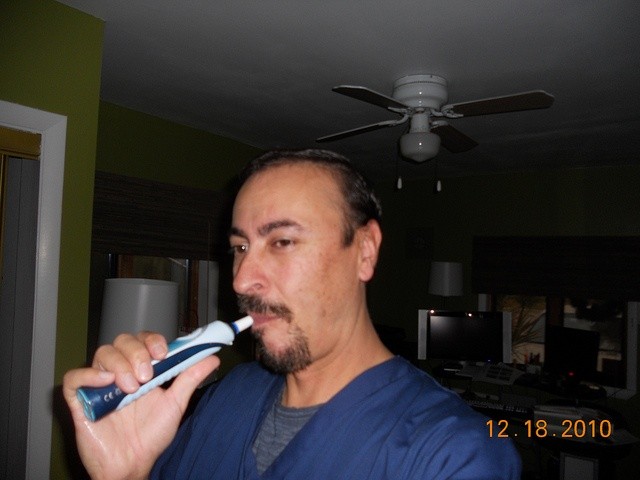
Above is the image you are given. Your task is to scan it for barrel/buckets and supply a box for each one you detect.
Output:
[96,278,179,349]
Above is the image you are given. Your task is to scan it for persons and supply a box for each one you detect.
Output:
[62,150,521,480]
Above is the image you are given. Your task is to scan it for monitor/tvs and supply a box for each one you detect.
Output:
[426,310,503,371]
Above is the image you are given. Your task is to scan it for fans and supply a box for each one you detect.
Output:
[315,75,555,160]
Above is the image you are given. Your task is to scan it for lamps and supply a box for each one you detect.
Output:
[427,260,466,309]
[397,116,442,163]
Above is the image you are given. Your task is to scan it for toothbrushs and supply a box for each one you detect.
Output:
[75,315,254,422]
